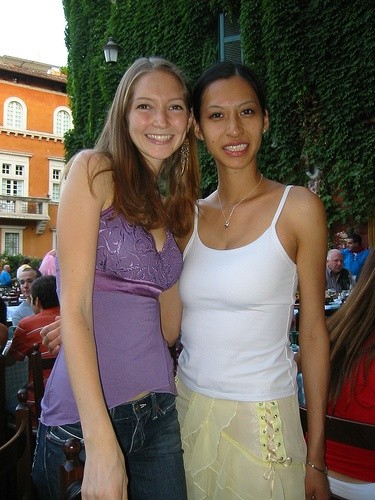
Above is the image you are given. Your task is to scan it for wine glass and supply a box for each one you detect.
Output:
[328,288,336,302]
[348,275,356,289]
[343,290,350,302]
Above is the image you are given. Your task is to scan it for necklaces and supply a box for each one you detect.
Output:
[215,169,264,229]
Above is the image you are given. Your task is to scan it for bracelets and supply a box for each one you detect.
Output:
[305,456,328,479]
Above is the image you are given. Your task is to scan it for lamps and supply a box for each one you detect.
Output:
[103,37,119,64]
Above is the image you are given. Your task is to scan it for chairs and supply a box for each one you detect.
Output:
[60,437,84,500]
[0,389,36,500]
[298,404,375,500]
[29,344,74,425]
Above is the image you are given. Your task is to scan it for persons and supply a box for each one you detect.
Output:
[0,255,10,274]
[37,248,58,278]
[0,297,9,351]
[291,247,375,500]
[39,315,64,355]
[340,234,370,282]
[16,264,32,282]
[157,58,332,500]
[27,54,201,500]
[0,264,18,289]
[325,248,356,292]
[9,267,45,328]
[3,273,62,430]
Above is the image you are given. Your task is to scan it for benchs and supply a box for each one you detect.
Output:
[0,286,21,307]
